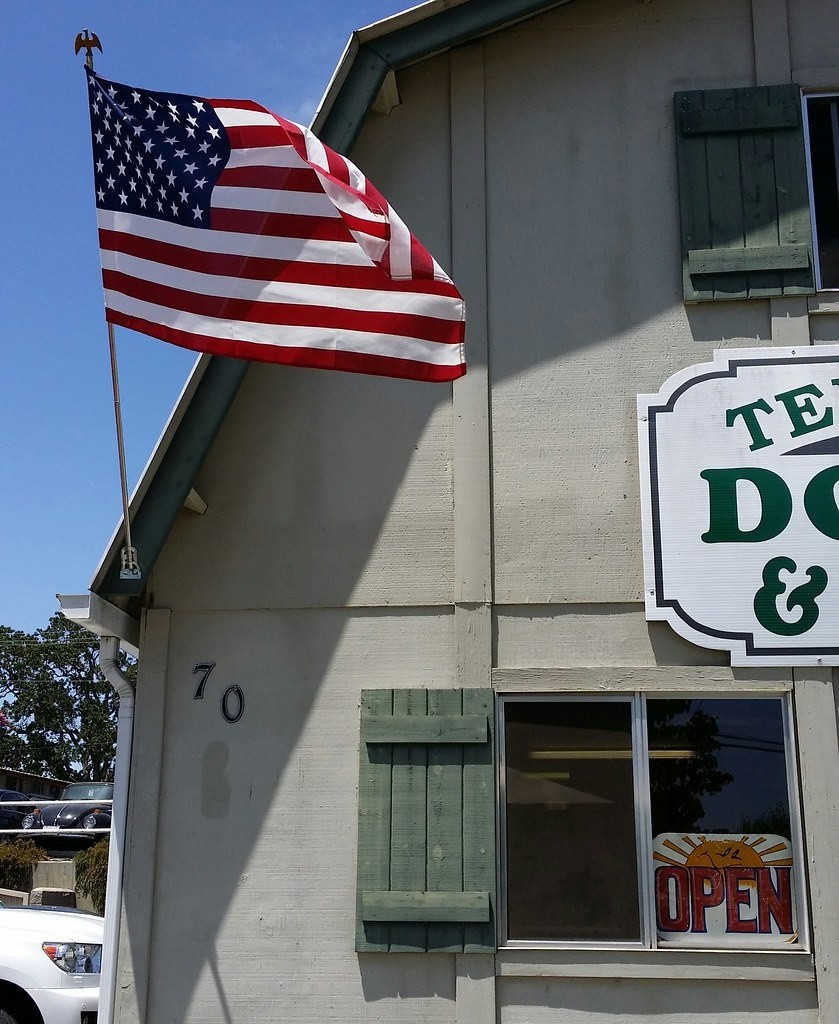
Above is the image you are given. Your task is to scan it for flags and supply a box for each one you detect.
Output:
[82,68,470,383]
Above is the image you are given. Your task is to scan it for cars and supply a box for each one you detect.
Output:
[0,899,108,1024]
[0,789,38,829]
[22,781,116,830]
